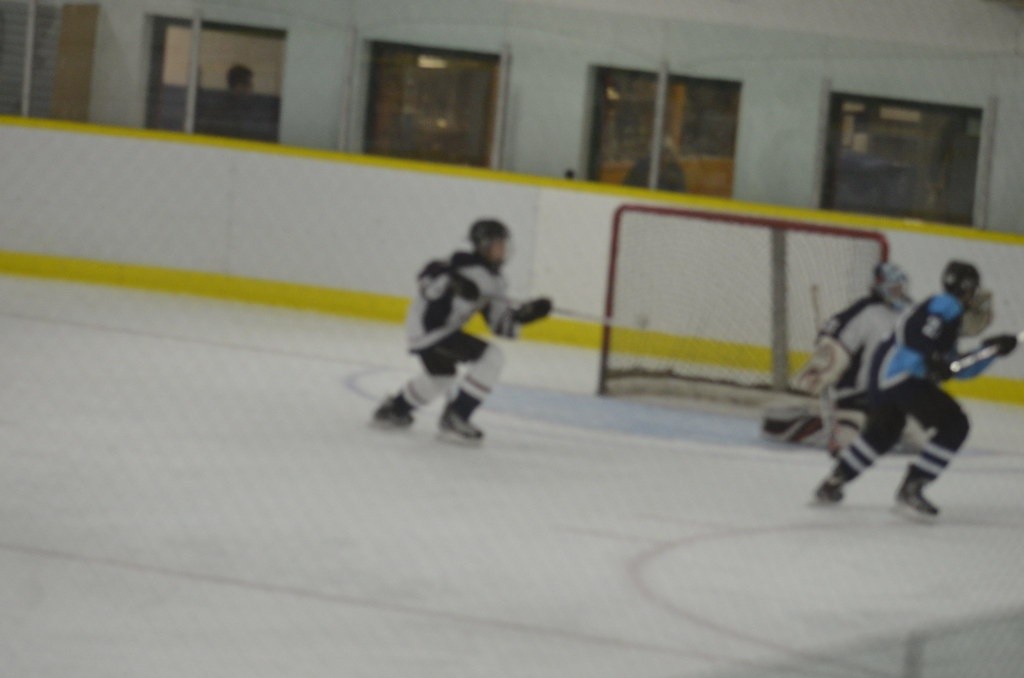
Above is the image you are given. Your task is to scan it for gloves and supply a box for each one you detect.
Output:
[984,335,1018,355]
[928,349,953,372]
[518,299,549,322]
[451,272,479,300]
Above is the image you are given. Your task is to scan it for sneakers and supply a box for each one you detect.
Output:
[816,485,843,505]
[375,405,412,427]
[438,409,483,445]
[896,490,937,518]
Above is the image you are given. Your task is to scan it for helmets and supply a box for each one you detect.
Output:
[471,221,504,253]
[943,261,979,296]
[871,263,907,304]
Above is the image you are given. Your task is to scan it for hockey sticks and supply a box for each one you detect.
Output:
[476,293,651,333]
[808,282,843,458]
[950,331,1024,373]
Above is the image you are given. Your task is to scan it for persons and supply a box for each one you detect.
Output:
[628,139,688,196]
[366,218,556,442]
[203,64,266,142]
[764,257,1019,517]
